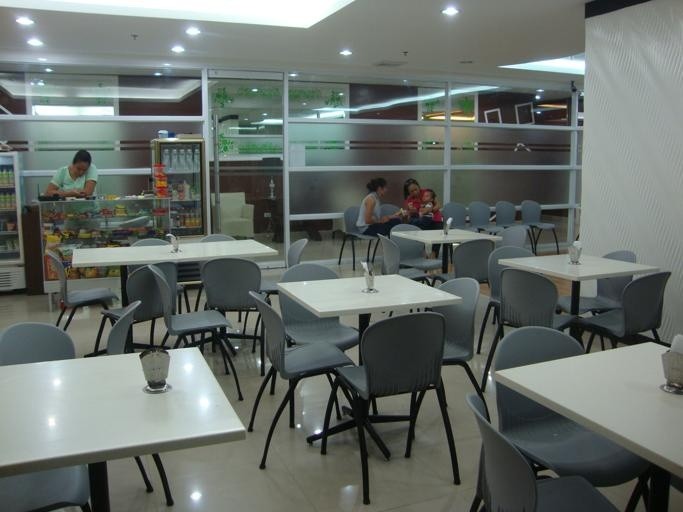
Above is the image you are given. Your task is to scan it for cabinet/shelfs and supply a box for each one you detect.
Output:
[38,193,170,282]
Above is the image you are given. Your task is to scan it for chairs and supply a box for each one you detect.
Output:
[378,204,400,217]
[199,258,272,352]
[147,263,244,401]
[477,247,541,355]
[248,288,396,469]
[337,204,380,271]
[430,240,496,288]
[479,269,584,391]
[390,224,443,283]
[125,237,190,314]
[465,393,622,512]
[556,249,638,353]
[91,261,176,353]
[577,271,671,353]
[374,232,433,287]
[494,201,533,254]
[467,201,503,237]
[105,300,142,356]
[491,324,657,512]
[242,238,309,353]
[277,261,363,430]
[318,312,462,505]
[0,321,95,512]
[402,275,492,485]
[47,247,118,332]
[440,201,478,263]
[519,199,559,253]
[193,234,246,323]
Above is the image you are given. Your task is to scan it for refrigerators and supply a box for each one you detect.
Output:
[149,136,207,240]
[0,151,27,291]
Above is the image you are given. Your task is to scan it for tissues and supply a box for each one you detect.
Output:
[567,240,583,265]
[359,261,378,293]
[443,218,452,233]
[166,233,182,253]
[659,333,683,395]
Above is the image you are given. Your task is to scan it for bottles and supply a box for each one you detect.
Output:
[194,148,201,171]
[171,207,201,228]
[0,168,3,184]
[171,148,178,171]
[5,192,11,209]
[186,148,194,171]
[178,148,186,171]
[3,168,9,184]
[11,193,16,209]
[0,191,5,208]
[8,168,14,185]
[163,148,170,170]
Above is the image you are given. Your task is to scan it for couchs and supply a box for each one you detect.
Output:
[210,190,255,238]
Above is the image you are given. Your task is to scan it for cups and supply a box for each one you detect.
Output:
[566,246,583,266]
[170,236,182,253]
[658,350,682,396]
[361,270,378,293]
[444,225,449,236]
[139,348,172,394]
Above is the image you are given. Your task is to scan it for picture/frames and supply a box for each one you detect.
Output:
[483,108,502,123]
[514,102,534,124]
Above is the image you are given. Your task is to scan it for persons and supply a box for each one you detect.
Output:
[399,178,444,259]
[401,189,436,219]
[46,149,99,203]
[355,177,402,239]
